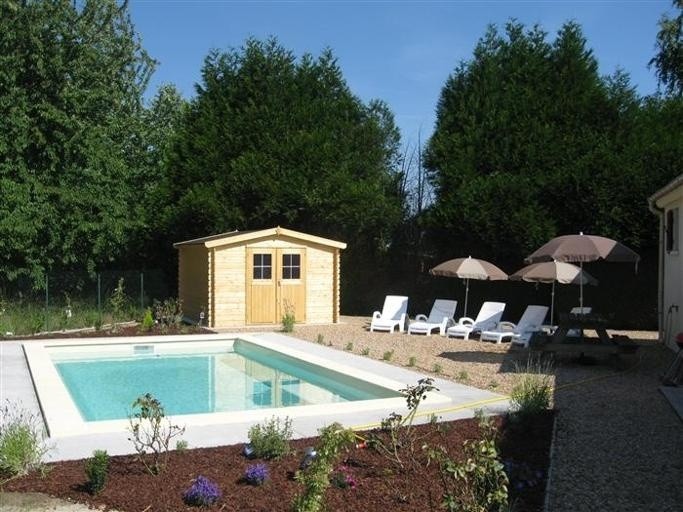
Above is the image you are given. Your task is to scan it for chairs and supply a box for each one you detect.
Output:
[369,294,550,348]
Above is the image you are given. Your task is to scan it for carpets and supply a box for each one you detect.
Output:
[658,384,683,422]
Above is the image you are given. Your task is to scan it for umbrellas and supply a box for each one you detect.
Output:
[506,258,599,325]
[519,231,641,333]
[427,255,510,317]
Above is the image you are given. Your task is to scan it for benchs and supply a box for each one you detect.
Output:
[527,330,641,371]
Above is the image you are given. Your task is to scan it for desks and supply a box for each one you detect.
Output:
[549,312,609,376]
